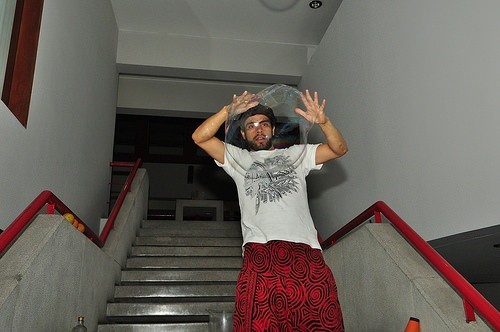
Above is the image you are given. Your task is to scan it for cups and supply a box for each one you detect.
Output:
[206,309,234,332]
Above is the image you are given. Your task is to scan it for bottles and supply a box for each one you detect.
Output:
[404,317,420,332]
[71,316,87,332]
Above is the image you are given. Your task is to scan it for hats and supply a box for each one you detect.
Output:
[237,104,275,129]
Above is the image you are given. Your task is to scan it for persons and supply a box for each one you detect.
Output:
[192,90,349,332]
